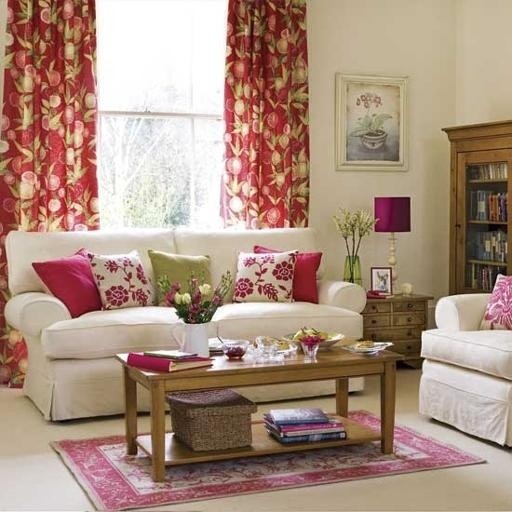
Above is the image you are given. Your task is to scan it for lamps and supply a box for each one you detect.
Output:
[375,196,410,295]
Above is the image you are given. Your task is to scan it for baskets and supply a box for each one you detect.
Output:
[165,389,257,452]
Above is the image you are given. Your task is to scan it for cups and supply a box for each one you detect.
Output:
[300,341,320,358]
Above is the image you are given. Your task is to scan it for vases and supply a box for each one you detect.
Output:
[361,131,387,150]
[343,255,363,286]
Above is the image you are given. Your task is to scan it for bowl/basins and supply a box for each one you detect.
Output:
[222,339,249,360]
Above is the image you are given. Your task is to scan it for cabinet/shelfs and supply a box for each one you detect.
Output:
[361,293,434,370]
[441,119,512,294]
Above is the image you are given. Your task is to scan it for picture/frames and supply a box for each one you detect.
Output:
[334,73,410,172]
[370,267,392,296]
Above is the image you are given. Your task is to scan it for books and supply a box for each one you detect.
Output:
[262,408,346,444]
[369,291,390,296]
[368,295,394,299]
[466,162,507,292]
[128,352,215,373]
[144,352,198,359]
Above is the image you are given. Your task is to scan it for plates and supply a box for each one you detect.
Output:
[281,332,345,349]
[343,342,393,355]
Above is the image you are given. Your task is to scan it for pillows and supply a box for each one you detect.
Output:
[480,274,512,330]
[232,250,298,304]
[88,250,154,310]
[147,249,213,306]
[32,248,103,319]
[253,246,322,304]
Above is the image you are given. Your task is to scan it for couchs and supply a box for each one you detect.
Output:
[4,229,367,421]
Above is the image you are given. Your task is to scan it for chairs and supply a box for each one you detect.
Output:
[419,292,512,448]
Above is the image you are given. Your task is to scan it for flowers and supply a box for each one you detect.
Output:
[157,269,233,323]
[349,93,392,136]
[332,206,374,283]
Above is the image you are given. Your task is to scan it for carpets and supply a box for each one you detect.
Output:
[48,410,487,512]
